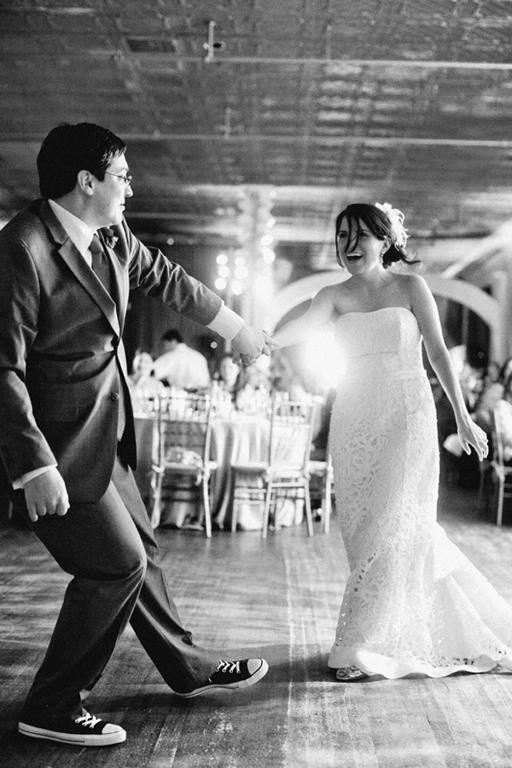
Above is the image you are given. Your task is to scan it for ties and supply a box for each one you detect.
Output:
[88,234,115,300]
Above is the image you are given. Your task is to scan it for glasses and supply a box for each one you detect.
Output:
[105,171,133,184]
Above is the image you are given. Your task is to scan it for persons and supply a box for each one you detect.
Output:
[248,203,512,680]
[125,329,289,413]
[433,347,509,457]
[1,119,285,748]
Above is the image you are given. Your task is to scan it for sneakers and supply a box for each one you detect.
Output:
[17,707,127,748]
[174,657,270,699]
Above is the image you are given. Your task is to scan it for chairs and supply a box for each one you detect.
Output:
[134,374,337,540]
[475,405,512,526]
